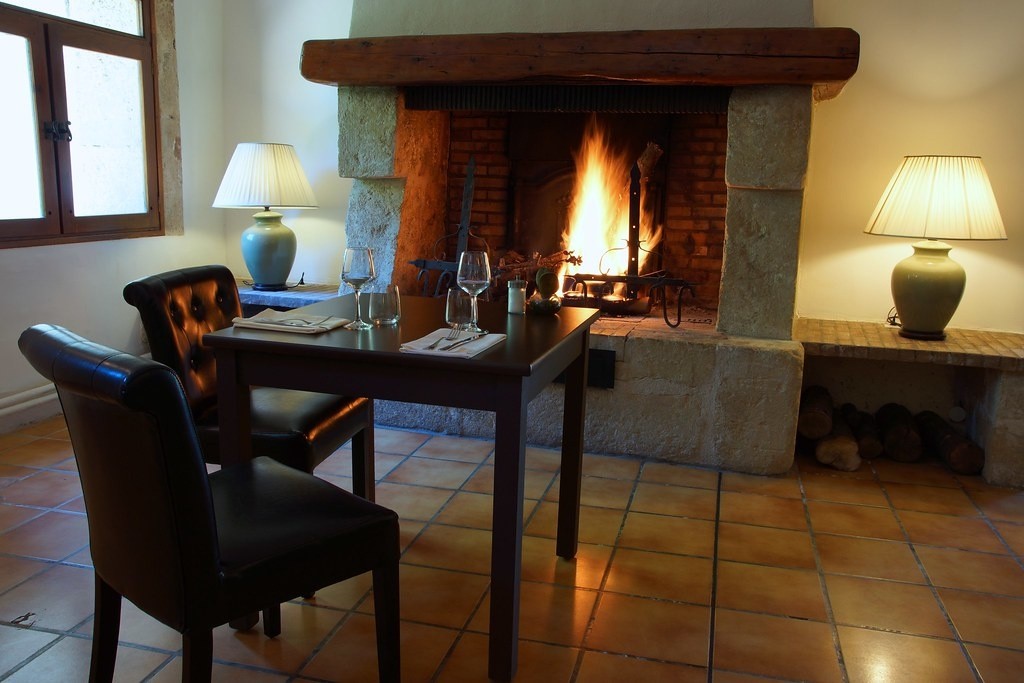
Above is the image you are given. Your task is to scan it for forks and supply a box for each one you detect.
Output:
[278,315,334,325]
[423,324,463,350]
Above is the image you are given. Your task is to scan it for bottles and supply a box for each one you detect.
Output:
[508,275,527,314]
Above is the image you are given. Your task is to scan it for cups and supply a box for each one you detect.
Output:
[368,284,401,325]
[445,287,473,329]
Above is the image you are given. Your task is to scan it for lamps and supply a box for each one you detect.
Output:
[212,142,318,293]
[863,154,1007,341]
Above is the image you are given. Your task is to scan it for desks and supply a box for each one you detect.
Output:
[202,293,601,683]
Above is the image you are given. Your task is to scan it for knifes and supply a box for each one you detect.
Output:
[438,333,487,352]
[255,321,325,328]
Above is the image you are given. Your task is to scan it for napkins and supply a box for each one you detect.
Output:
[400,329,508,360]
[232,308,350,334]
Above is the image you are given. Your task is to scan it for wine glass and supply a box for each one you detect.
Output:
[341,247,375,330]
[456,251,491,334]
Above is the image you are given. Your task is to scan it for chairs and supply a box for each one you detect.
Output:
[17,324,400,683]
[122,265,378,599]
